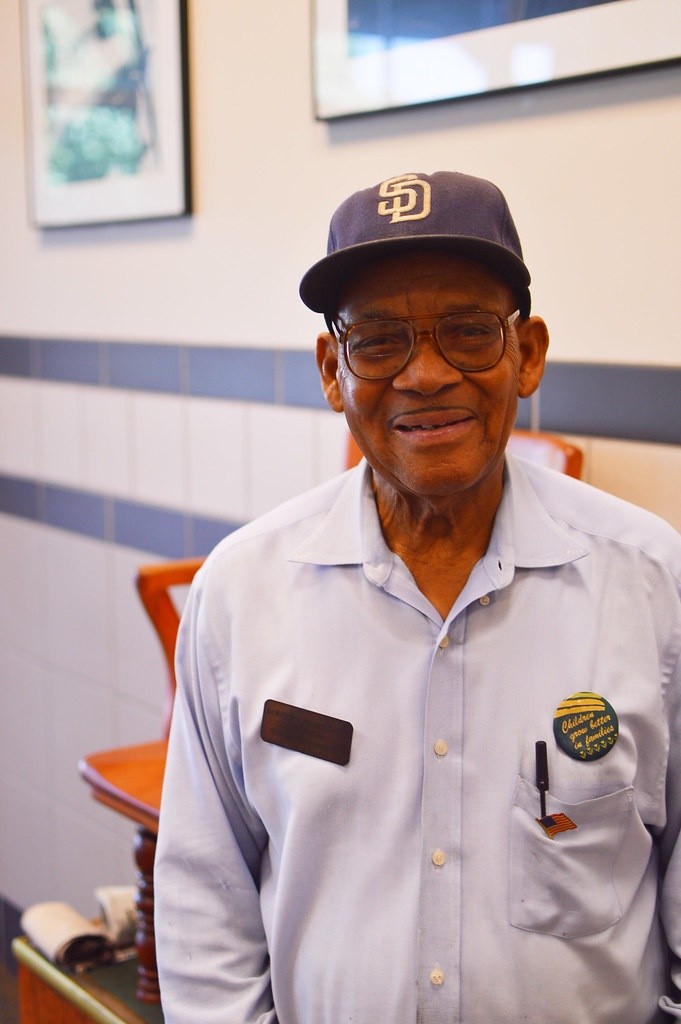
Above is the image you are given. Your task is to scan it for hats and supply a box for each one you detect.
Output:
[300,172,531,313]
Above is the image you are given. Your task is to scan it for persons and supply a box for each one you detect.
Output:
[154,172,680,1024]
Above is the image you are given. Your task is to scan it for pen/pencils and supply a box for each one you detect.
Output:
[534,740,552,820]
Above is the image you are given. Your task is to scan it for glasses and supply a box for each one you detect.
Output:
[330,306,520,380]
